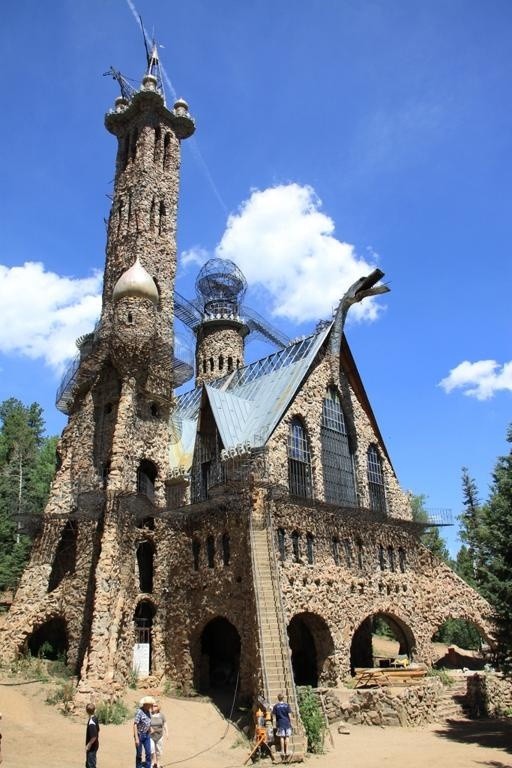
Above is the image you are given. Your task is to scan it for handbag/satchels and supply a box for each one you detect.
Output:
[139,734,147,742]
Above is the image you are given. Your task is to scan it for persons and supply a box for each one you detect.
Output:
[271,694,294,754]
[85,702,99,768]
[149,699,169,768]
[132,696,153,768]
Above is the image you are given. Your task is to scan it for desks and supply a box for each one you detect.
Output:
[355,668,393,691]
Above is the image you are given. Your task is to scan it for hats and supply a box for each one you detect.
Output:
[140,696,155,704]
